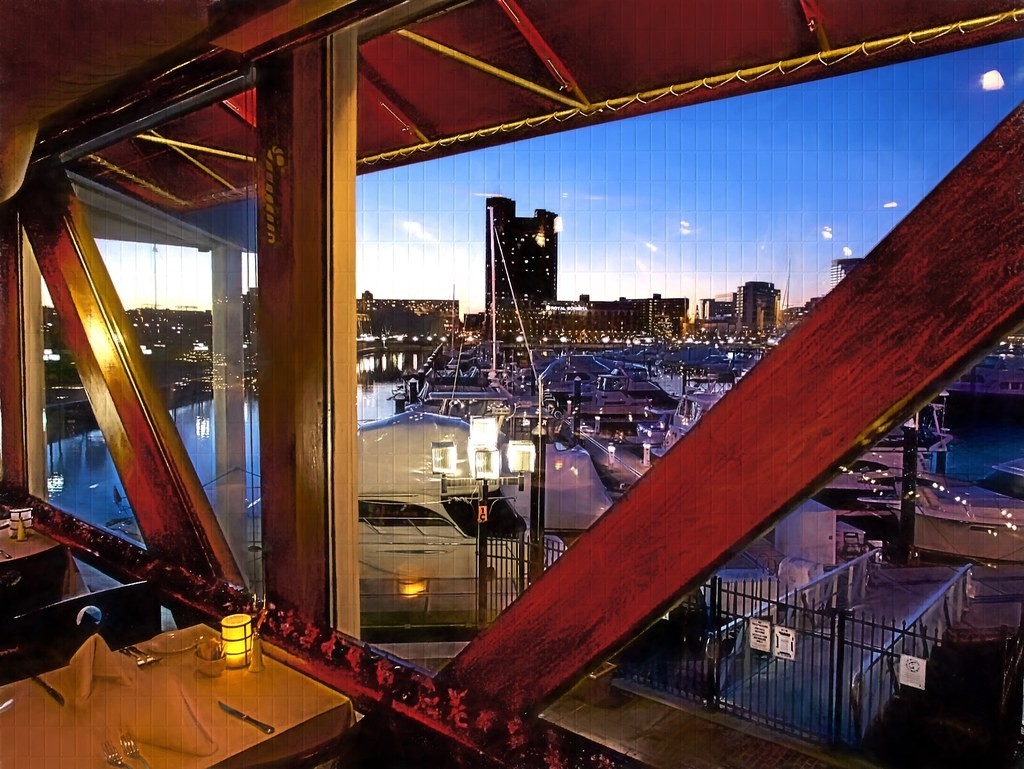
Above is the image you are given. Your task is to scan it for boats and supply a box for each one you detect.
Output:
[528,338,1024,564]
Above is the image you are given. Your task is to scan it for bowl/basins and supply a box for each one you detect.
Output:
[194,651,226,677]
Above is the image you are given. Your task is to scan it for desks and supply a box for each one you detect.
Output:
[0,622,377,769]
[0,518,91,602]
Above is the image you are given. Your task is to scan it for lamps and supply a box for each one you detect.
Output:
[219,613,254,670]
[9,507,33,536]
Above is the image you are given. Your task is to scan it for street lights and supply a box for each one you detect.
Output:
[431,414,537,626]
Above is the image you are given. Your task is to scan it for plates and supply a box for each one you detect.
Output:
[147,634,197,654]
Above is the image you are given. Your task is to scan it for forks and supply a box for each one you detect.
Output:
[119,648,151,666]
[102,741,134,769]
[129,646,162,663]
[119,735,151,769]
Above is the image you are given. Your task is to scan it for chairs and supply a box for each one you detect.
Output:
[0,543,66,619]
[14,577,161,672]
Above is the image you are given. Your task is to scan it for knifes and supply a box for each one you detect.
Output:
[218,701,274,733]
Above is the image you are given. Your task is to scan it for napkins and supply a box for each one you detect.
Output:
[70,632,140,713]
[126,683,219,755]
